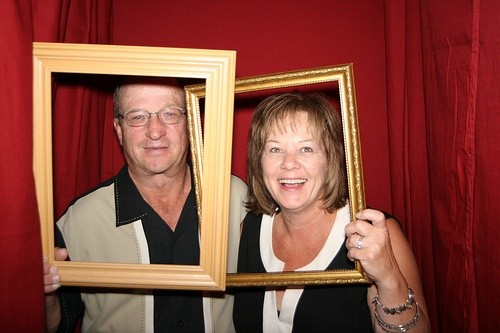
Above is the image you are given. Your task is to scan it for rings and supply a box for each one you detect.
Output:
[355,235,365,249]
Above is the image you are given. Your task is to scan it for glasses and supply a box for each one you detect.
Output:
[116,106,187,128]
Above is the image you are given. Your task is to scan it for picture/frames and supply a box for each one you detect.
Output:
[31,42,237,292]
[182,62,374,288]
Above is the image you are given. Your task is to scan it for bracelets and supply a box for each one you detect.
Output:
[371,285,420,333]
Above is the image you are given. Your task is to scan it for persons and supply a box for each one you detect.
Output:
[43,75,257,333]
[233,90,432,332]
